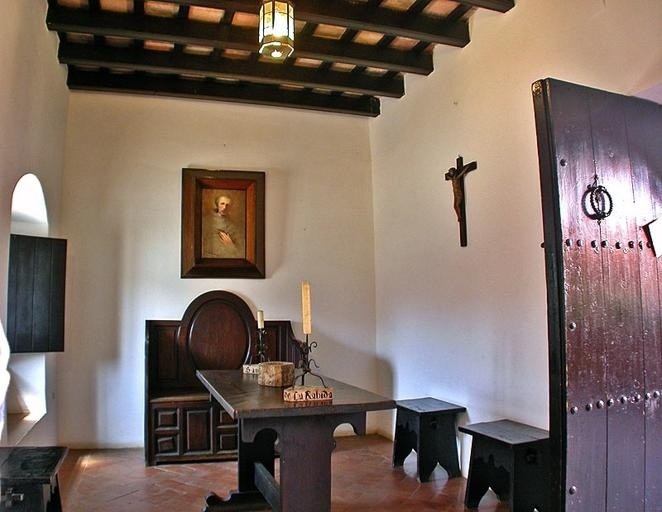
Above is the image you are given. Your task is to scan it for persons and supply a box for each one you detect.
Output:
[444,163,473,225]
[200,187,249,262]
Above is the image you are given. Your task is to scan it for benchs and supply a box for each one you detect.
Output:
[142,292,302,467]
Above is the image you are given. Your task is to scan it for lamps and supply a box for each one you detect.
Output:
[258,0,295,64]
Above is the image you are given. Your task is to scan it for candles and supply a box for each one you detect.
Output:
[301,279,312,334]
[256,311,265,330]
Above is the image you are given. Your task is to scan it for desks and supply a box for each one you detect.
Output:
[195,368,396,512]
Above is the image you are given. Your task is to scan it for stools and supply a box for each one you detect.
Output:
[392,397,467,483]
[0,446,70,512]
[458,420,552,512]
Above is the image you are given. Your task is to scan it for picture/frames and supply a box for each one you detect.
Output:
[181,168,265,279]
